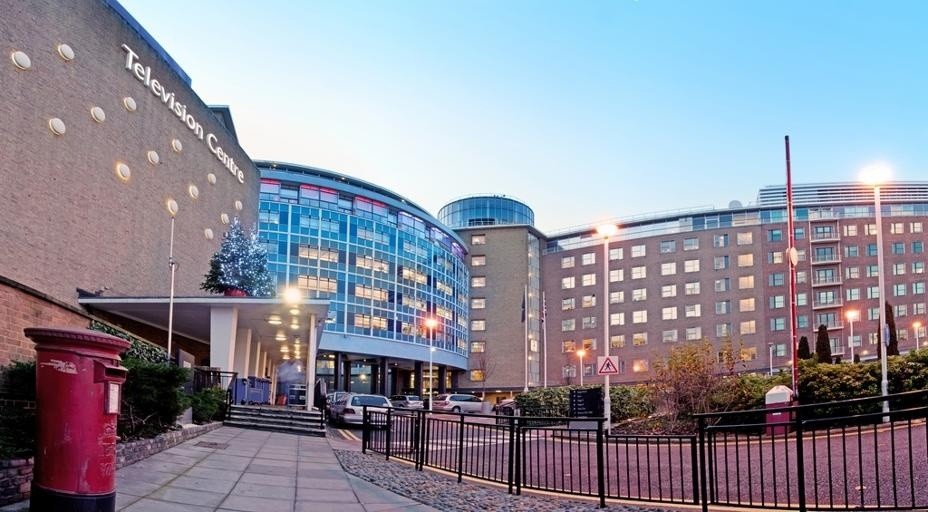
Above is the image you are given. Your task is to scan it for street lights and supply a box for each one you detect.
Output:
[865,170,891,422]
[596,222,619,436]
[913,321,923,351]
[424,318,438,420]
[575,349,586,386]
[846,310,860,365]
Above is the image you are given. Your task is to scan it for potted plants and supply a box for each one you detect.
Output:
[199,213,267,297]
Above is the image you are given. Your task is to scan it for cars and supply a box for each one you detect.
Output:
[326,392,519,431]
